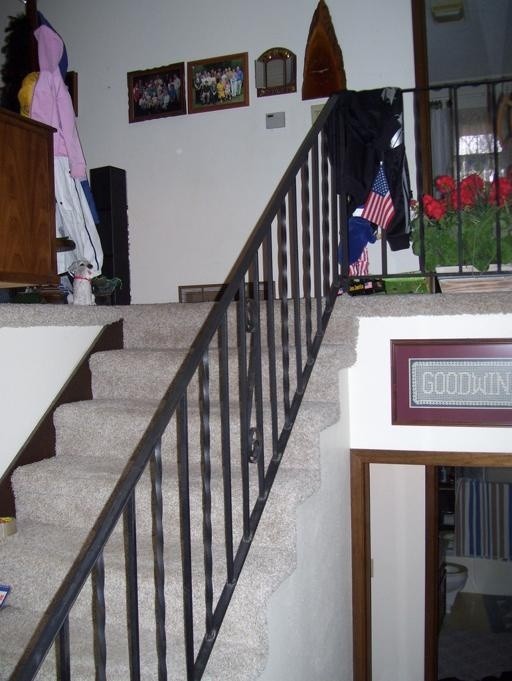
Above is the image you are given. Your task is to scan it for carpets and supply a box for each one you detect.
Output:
[486,595,512,634]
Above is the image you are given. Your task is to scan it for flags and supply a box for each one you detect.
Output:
[361,165,395,231]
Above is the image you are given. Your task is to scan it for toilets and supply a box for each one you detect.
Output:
[445,562,468,615]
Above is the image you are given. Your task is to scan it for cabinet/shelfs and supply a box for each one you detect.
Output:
[439,467,455,528]
[0,108,61,288]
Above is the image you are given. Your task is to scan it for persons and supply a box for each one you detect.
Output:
[194,66,243,103]
[133,74,181,114]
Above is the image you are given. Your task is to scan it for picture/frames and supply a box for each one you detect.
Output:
[65,71,78,117]
[187,52,249,113]
[127,62,186,123]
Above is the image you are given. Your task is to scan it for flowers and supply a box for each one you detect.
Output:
[409,165,512,270]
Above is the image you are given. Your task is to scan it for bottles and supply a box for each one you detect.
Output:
[440,466,448,483]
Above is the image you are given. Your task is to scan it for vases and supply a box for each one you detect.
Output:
[435,263,512,294]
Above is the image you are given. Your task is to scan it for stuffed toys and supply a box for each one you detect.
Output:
[67,259,97,305]
